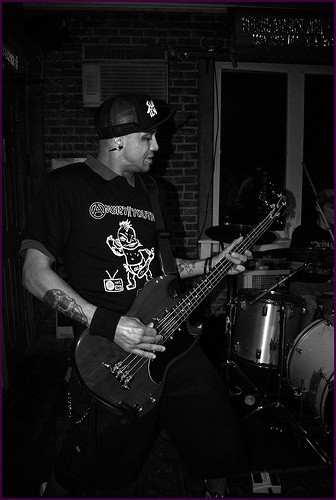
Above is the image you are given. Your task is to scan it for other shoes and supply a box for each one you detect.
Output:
[205,480,230,498]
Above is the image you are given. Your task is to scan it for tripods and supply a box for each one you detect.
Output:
[215,231,335,467]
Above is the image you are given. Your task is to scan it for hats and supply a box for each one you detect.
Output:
[97,94,176,137]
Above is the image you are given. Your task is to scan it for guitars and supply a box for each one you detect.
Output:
[70,181,289,419]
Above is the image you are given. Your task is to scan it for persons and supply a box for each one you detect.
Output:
[18,94,252,498]
[287,189,334,337]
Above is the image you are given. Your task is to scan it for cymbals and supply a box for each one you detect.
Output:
[205,224,277,246]
[262,246,334,260]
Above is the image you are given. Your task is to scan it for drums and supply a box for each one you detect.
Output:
[228,288,307,370]
[285,317,335,433]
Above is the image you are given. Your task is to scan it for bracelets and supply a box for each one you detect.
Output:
[90,306,121,342]
[209,256,213,271]
[204,257,209,274]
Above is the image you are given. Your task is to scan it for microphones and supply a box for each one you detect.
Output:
[295,259,314,271]
